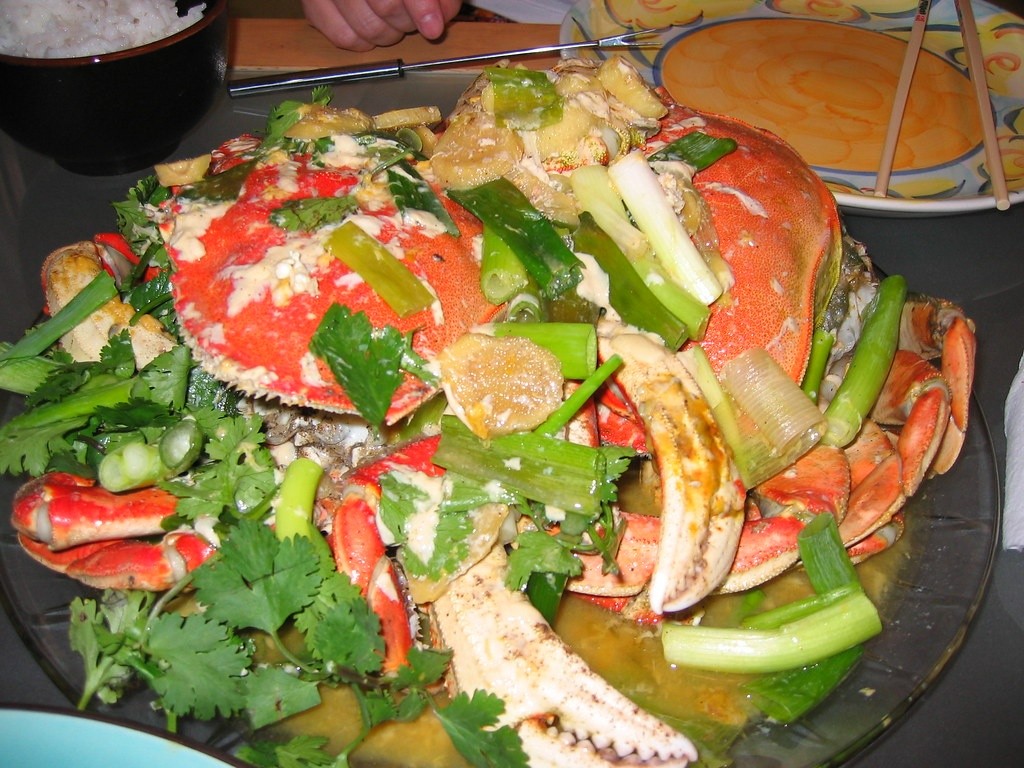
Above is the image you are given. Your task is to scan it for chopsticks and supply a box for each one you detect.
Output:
[870,0,1010,211]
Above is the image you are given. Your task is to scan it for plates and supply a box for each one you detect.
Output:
[0,121,1001,767]
[558,0,1024,219]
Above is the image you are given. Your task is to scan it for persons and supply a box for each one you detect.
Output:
[301,1,580,53]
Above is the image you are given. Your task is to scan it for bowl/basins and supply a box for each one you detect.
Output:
[0,0,229,181]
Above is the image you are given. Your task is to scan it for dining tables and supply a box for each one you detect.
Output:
[0,20,1024,768]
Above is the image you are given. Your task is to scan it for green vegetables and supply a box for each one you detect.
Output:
[0,56,979,768]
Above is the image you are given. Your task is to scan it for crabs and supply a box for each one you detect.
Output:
[10,56,978,768]
